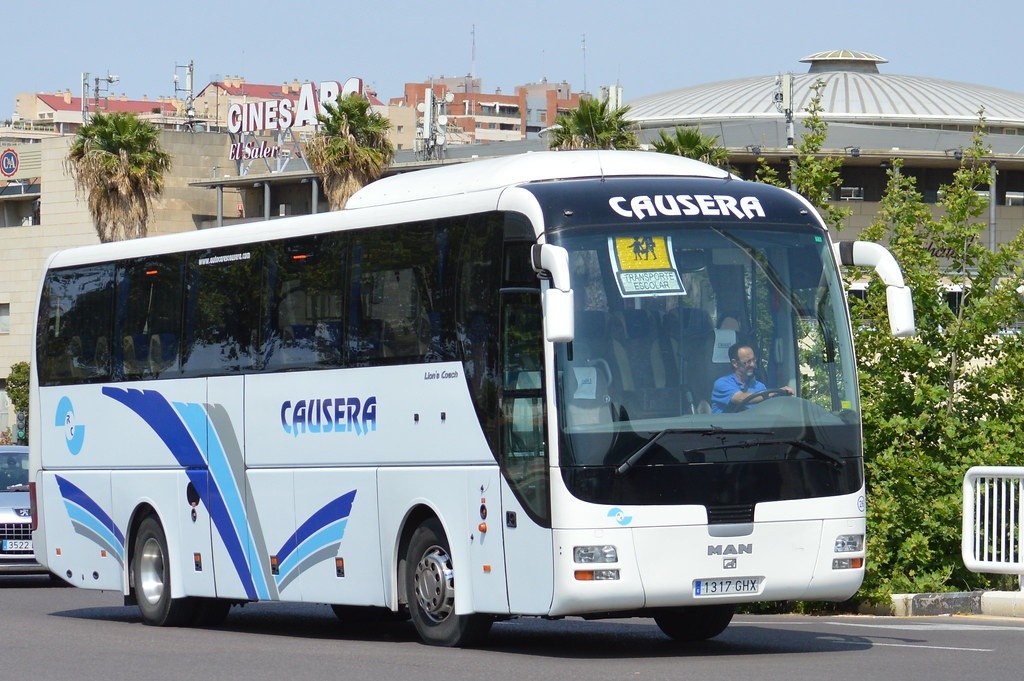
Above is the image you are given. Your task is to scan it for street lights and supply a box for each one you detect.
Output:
[953,148,997,293]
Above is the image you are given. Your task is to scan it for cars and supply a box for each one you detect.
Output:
[0,443,75,585]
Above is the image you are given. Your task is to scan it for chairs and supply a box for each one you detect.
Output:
[564,305,744,426]
[46,319,399,381]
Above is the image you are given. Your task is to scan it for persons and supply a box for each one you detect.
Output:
[711,342,795,413]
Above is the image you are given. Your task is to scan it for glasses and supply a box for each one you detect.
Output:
[736,357,757,366]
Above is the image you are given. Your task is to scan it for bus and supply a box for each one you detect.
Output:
[27,148,917,645]
